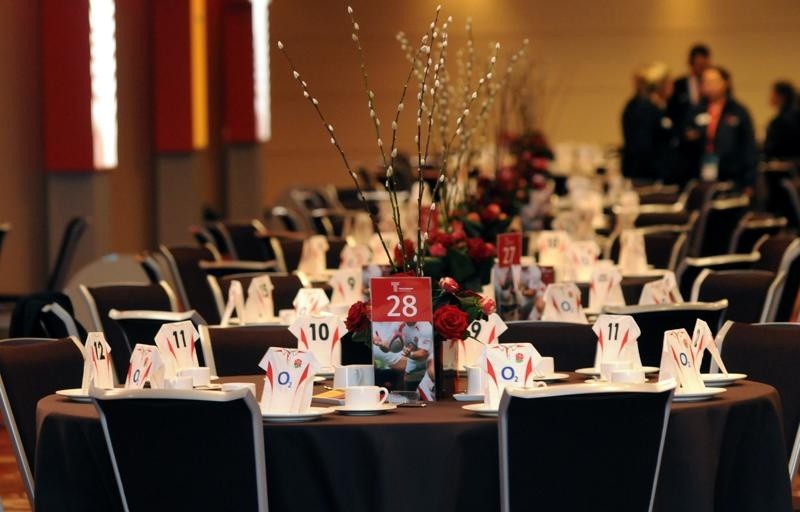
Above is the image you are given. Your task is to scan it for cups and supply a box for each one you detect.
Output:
[165,377,193,391]
[221,382,256,401]
[176,367,211,386]
[540,357,554,376]
[344,386,388,409]
[333,364,375,388]
[466,367,482,395]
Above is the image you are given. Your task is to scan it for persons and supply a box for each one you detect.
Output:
[688,64,758,188]
[667,44,720,185]
[372,323,432,389]
[761,79,800,165]
[620,61,680,188]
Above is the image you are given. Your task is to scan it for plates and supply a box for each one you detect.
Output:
[699,373,748,388]
[532,373,569,381]
[55,389,124,403]
[461,404,499,416]
[453,393,484,401]
[331,405,398,417]
[575,366,660,375]
[260,407,335,424]
[672,388,727,402]
